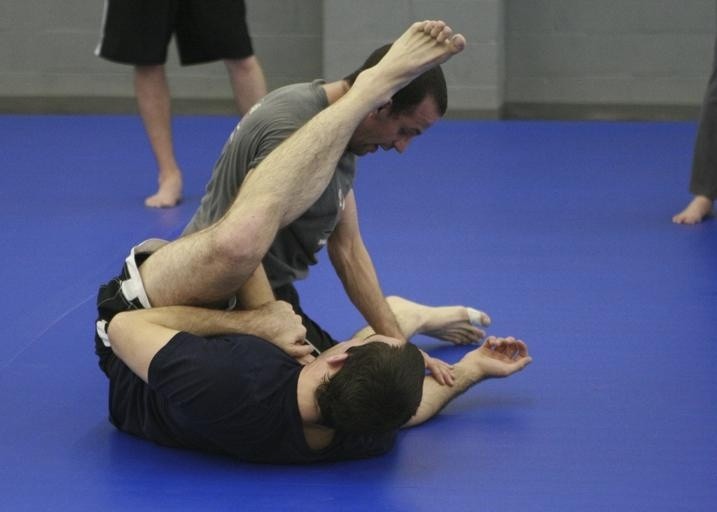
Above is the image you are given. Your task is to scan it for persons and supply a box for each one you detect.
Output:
[673,32,716,224]
[97,19,534,465]
[93,0,268,209]
[171,40,458,385]
